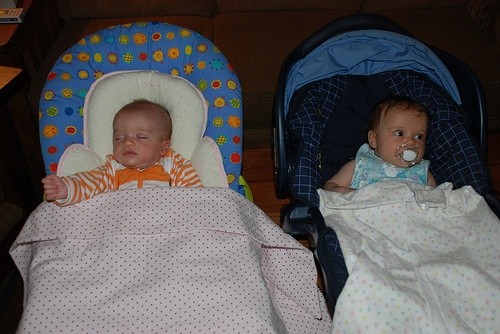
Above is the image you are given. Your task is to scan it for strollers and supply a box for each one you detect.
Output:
[272,14,500,334]
[17,22,283,334]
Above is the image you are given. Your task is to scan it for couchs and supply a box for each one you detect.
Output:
[55,0,500,180]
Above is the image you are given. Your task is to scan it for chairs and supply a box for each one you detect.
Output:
[37,20,243,195]
[286,70,491,205]
